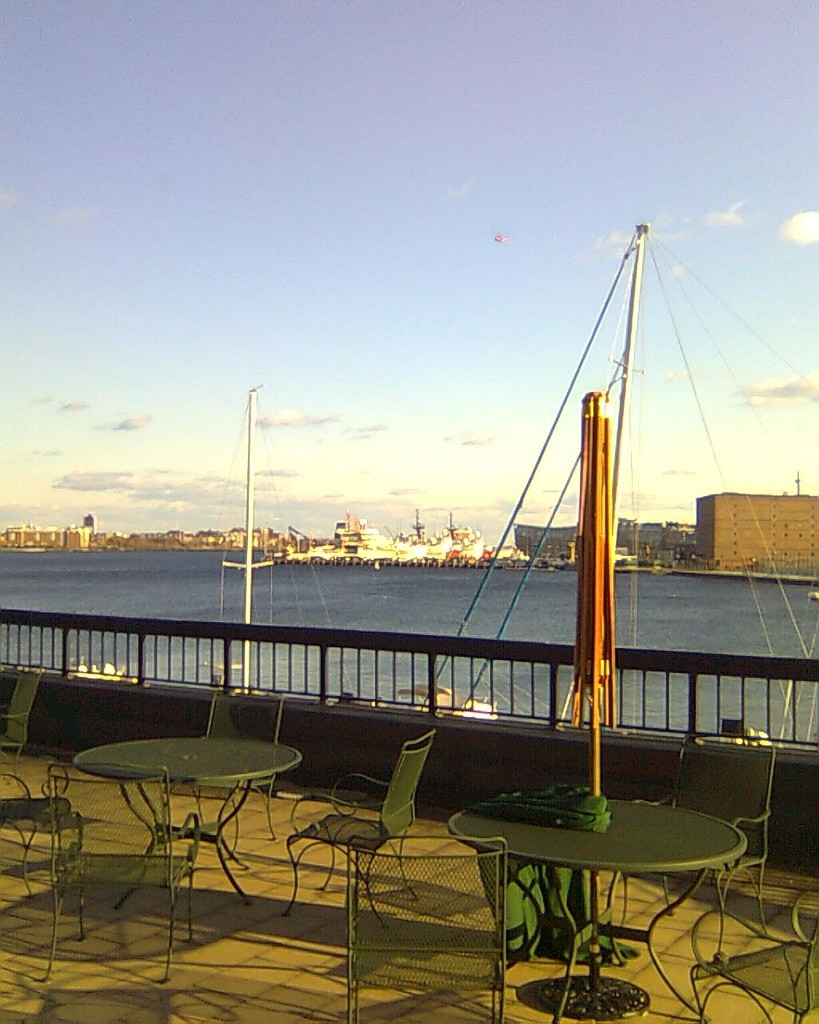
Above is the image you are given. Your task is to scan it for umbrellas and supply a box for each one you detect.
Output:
[571,391,616,796]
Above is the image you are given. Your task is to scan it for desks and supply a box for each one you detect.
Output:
[71,738,304,909]
[445,793,747,1024]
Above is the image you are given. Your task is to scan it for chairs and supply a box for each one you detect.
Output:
[152,689,285,862]
[616,731,778,958]
[0,667,88,908]
[280,727,437,931]
[341,833,507,1024]
[33,761,200,987]
[689,886,819,1024]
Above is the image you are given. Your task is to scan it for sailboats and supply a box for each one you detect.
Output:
[357,223,818,754]
